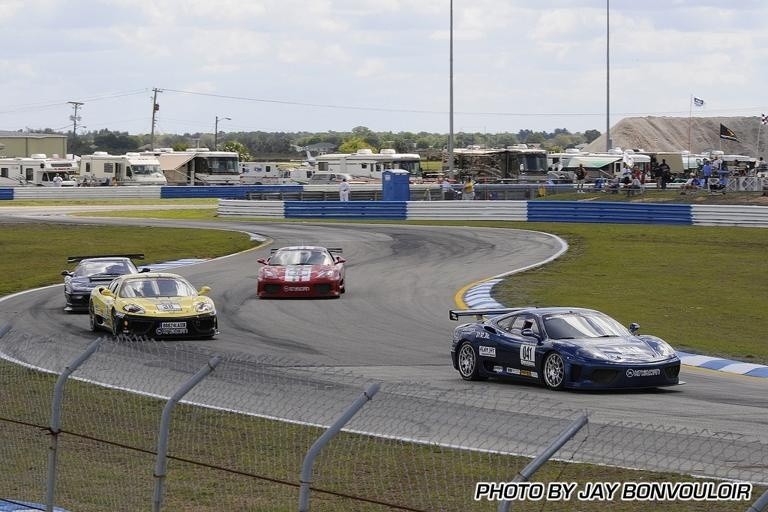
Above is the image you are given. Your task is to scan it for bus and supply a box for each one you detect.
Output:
[345,147,423,185]
[441,142,549,185]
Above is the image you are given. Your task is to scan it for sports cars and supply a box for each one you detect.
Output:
[85,268,223,340]
[255,247,351,299]
[60,252,150,313]
[446,304,684,392]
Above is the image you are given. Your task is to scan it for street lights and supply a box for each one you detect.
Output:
[214,114,233,151]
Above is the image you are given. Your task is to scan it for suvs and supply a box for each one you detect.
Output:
[546,171,575,185]
[308,173,352,185]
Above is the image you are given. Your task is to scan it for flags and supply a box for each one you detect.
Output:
[761,113,768,126]
[693,97,705,107]
[720,122,741,144]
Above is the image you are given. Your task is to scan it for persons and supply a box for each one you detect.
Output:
[574,163,587,193]
[660,159,670,189]
[52,173,63,187]
[601,165,644,198]
[338,178,350,202]
[441,178,451,201]
[654,163,663,191]
[463,176,475,200]
[680,156,767,195]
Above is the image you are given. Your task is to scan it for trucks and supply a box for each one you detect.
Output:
[1,147,242,186]
[274,169,314,184]
